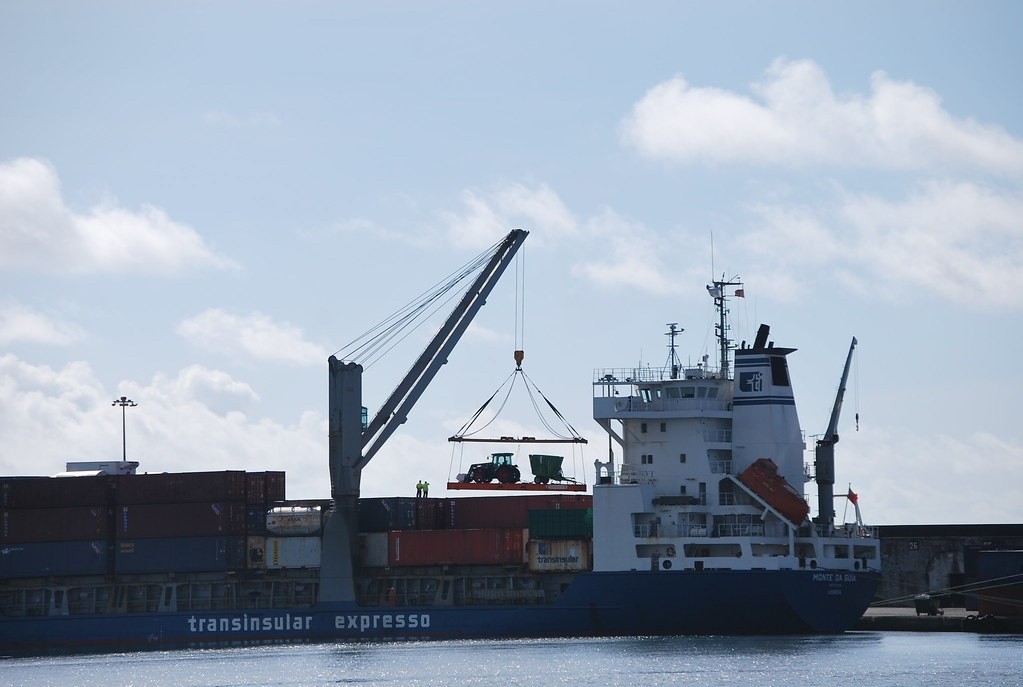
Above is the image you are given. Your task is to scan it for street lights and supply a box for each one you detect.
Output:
[112,397,137,461]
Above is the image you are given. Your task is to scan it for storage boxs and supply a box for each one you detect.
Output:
[0,470,593,581]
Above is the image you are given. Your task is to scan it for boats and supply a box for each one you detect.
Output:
[0,228,885,660]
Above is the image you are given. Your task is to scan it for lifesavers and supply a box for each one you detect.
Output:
[666,547,674,557]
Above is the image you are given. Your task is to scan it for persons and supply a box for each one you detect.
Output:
[416,480,423,497]
[423,481,429,498]
[844,523,848,538]
[389,587,395,606]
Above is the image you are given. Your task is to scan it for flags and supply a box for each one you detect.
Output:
[735,289,744,297]
[848,488,858,505]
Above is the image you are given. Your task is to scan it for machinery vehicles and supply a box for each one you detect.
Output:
[457,453,520,483]
